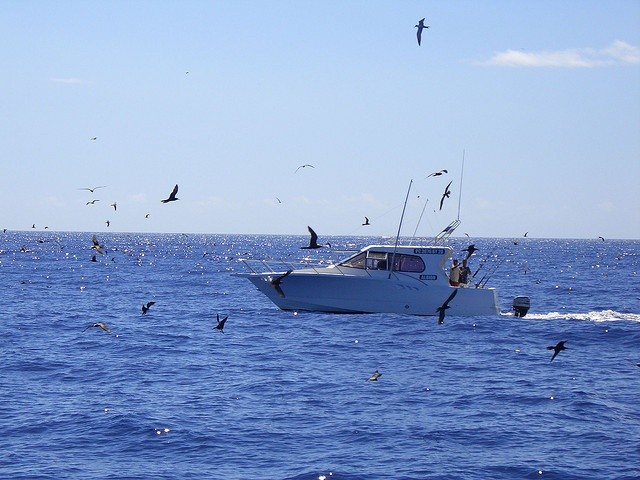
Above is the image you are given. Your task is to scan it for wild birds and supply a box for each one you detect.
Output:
[361,216,371,226]
[545,339,572,362]
[439,180,453,211]
[436,288,458,325]
[77,186,102,193]
[105,220,111,227]
[523,231,529,238]
[161,183,180,204]
[365,369,382,383]
[88,234,106,255]
[299,225,332,250]
[85,199,100,205]
[141,301,157,316]
[31,223,36,229]
[90,255,98,262]
[413,17,432,48]
[266,269,295,299]
[598,236,605,242]
[211,313,229,334]
[295,164,316,172]
[110,201,118,211]
[461,242,479,260]
[426,169,449,178]
[82,322,111,335]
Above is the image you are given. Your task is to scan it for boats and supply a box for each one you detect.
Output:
[229,244,531,318]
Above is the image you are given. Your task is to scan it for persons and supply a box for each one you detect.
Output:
[449,258,466,287]
[458,259,472,288]
